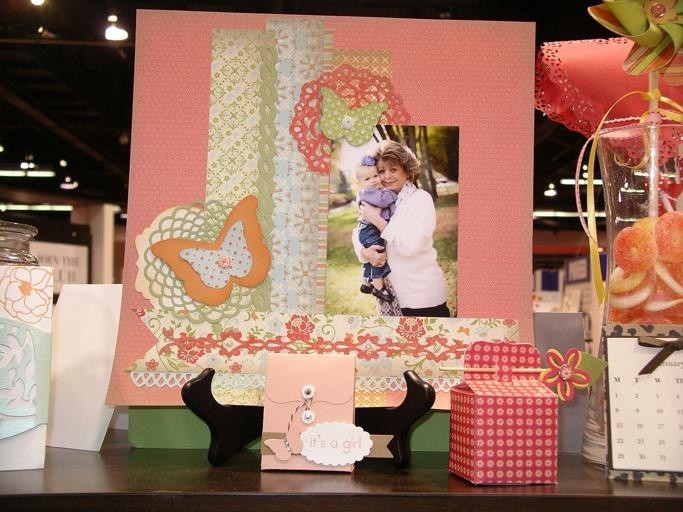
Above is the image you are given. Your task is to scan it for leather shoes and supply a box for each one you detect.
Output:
[372,287,394,301]
[361,285,379,296]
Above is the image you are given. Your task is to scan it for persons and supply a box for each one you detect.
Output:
[354,157,399,302]
[352,140,451,318]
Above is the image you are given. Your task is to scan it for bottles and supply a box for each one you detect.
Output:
[0,222,38,265]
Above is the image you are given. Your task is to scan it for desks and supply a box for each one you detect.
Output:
[0,426,683,507]
